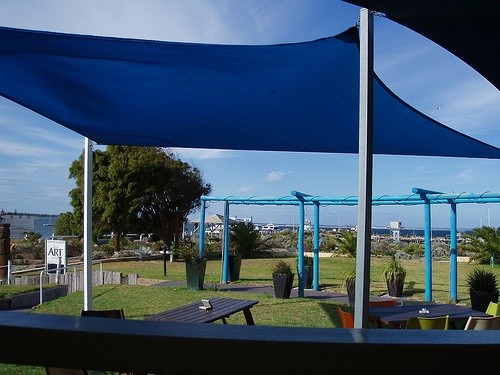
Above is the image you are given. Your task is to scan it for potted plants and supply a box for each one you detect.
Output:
[381,256,406,297]
[178,245,207,291]
[297,256,313,289]
[465,266,500,313]
[272,260,295,299]
[343,263,356,303]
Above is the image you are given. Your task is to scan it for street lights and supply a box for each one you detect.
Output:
[330,210,341,230]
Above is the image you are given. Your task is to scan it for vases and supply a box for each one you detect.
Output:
[227,255,242,281]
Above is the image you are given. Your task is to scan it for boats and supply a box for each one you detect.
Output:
[204,212,356,243]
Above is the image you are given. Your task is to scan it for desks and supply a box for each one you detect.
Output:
[369,303,494,329]
[146,299,259,326]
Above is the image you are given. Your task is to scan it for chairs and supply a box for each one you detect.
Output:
[464,316,500,331]
[338,307,354,328]
[486,301,500,317]
[405,316,449,329]
